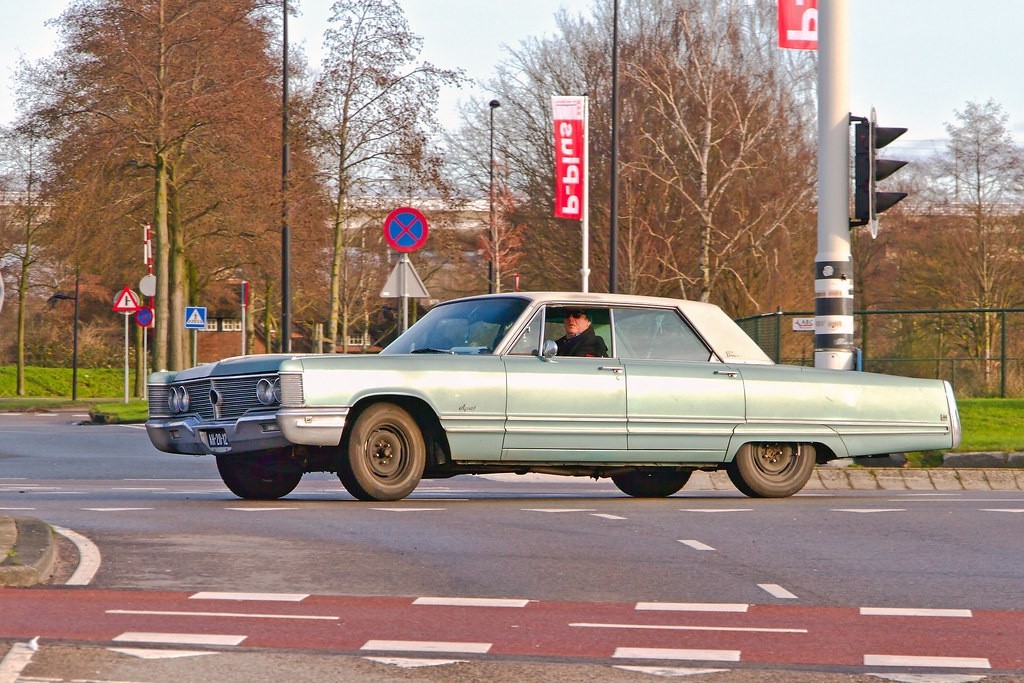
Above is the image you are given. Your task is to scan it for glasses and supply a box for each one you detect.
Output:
[564,311,586,318]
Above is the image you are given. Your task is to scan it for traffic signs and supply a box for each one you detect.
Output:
[182,307,206,329]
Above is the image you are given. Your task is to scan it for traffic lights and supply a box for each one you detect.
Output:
[853,107,908,223]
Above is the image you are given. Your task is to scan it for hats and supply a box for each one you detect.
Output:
[583,309,592,323]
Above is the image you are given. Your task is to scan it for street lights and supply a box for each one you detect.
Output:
[47,295,76,402]
[489,99,501,291]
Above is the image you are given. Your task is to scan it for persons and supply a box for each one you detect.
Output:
[552,308,610,359]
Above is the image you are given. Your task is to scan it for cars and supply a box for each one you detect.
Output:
[144,291,961,503]
